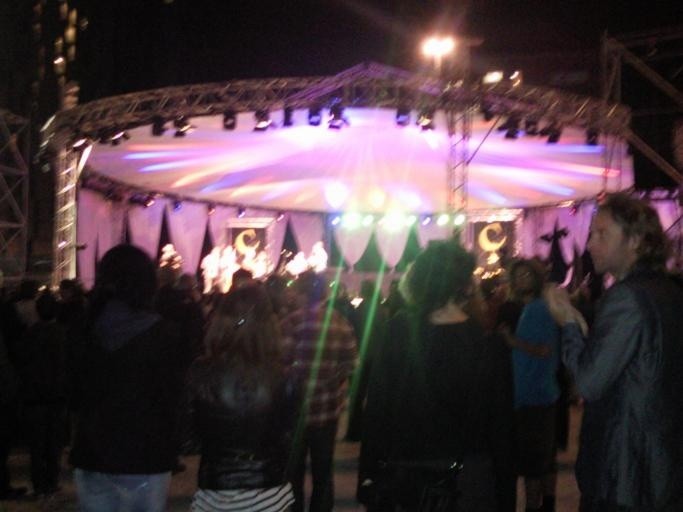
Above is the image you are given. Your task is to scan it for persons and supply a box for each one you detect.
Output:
[1,194,683,511]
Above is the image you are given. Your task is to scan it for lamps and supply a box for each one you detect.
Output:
[307,104,327,126]
[172,114,200,138]
[251,108,279,133]
[101,125,129,145]
[282,106,295,126]
[479,105,568,143]
[416,106,438,131]
[327,106,351,129]
[222,111,239,130]
[151,114,169,139]
[583,110,599,147]
[396,105,416,124]
[68,119,90,150]
